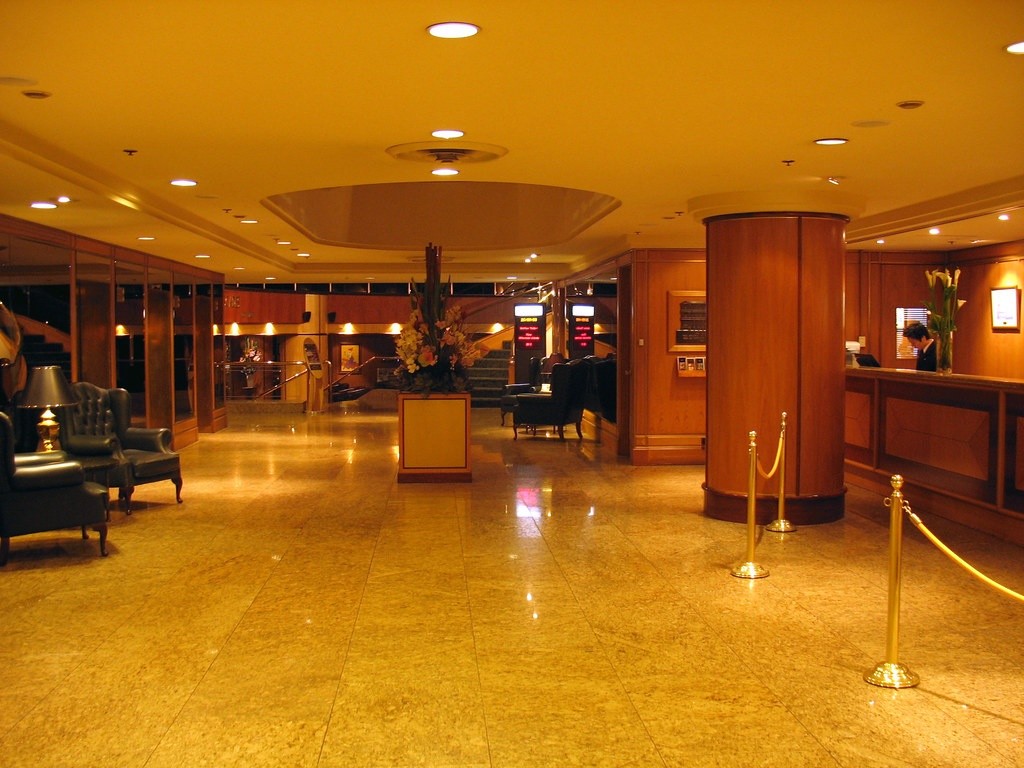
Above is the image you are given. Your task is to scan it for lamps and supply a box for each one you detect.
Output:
[116,324,130,337]
[16,366,85,452]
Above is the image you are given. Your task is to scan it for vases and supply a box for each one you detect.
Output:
[935,332,953,376]
[241,387,256,400]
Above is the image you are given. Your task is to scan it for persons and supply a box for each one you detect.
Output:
[903,323,951,372]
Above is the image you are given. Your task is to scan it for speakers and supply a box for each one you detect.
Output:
[328,311,336,322]
[303,311,311,323]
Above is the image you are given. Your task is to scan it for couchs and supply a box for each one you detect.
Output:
[512,358,584,443]
[500,356,549,427]
[54,382,183,515]
[0,411,110,569]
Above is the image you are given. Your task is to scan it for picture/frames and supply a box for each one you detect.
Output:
[338,341,362,375]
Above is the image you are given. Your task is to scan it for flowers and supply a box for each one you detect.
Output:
[393,274,490,394]
[239,338,262,387]
[923,268,966,333]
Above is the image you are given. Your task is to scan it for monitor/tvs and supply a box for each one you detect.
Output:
[853,353,881,368]
